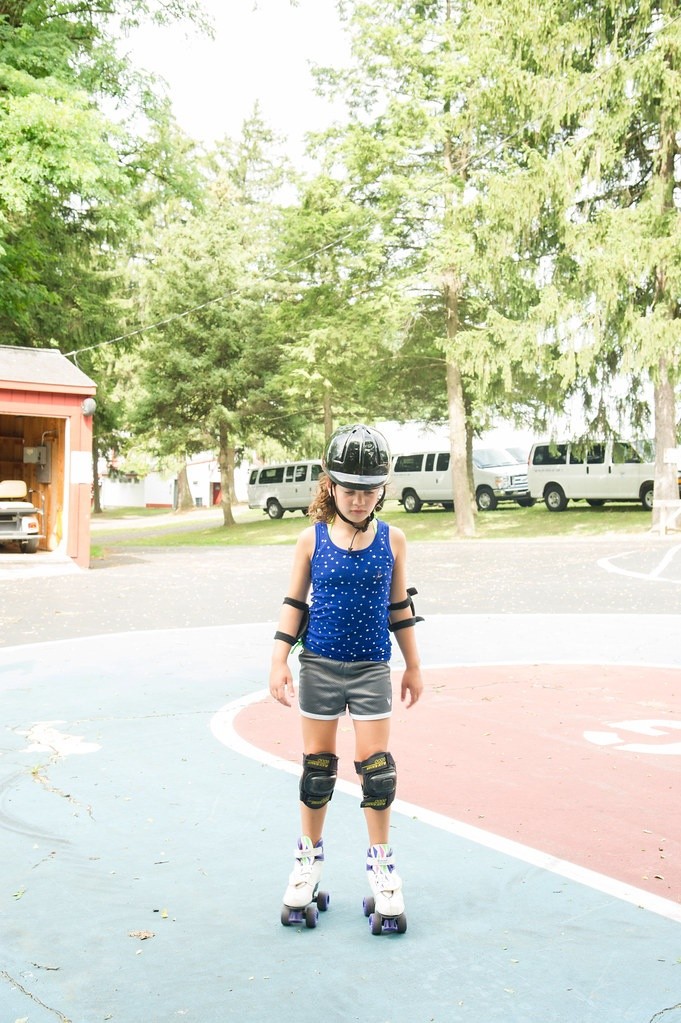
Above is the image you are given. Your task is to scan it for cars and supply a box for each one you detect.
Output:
[0,479,48,556]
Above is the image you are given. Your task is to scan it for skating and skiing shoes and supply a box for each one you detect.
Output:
[281,835,330,928]
[363,844,407,935]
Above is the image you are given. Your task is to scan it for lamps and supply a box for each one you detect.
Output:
[82,398,97,415]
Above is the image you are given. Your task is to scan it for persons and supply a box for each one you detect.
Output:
[269,424,424,936]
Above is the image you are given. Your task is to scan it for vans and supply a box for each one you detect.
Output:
[383,450,538,510]
[528,439,681,512]
[247,460,326,519]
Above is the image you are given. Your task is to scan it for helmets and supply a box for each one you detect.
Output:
[322,423,391,490]
